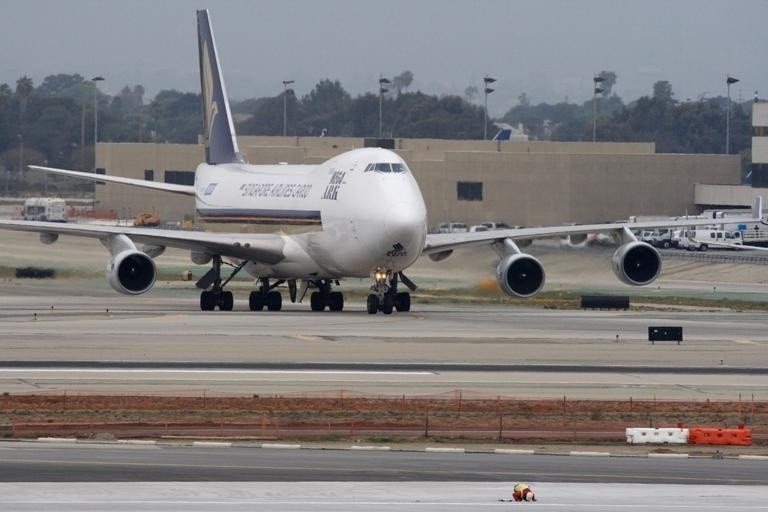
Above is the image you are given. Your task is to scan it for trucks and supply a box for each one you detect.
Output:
[585,228,742,252]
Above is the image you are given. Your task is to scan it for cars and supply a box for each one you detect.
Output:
[437,222,522,232]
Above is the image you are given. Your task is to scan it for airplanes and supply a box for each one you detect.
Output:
[0,8,764,318]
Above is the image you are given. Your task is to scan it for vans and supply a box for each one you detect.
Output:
[22,197,66,222]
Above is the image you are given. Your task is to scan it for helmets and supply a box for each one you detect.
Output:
[526,491,534,500]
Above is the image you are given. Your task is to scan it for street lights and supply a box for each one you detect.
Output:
[17,134,24,181]
